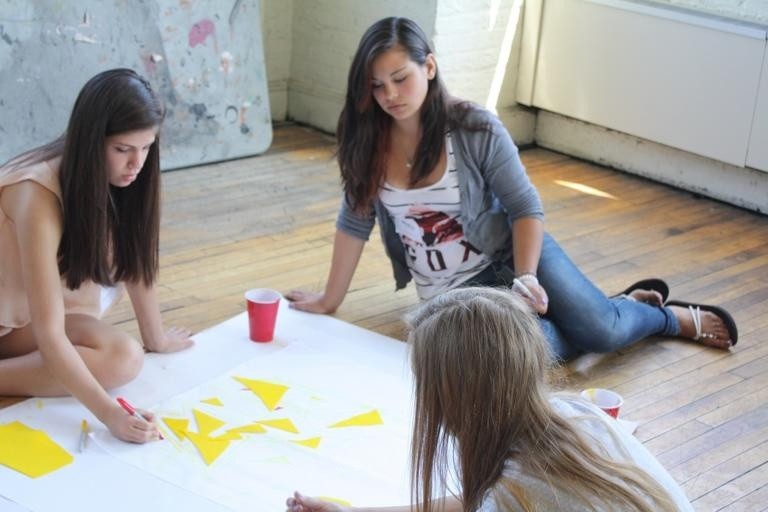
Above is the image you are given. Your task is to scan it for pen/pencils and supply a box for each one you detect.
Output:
[80,419,89,453]
[117,397,167,441]
[502,265,538,306]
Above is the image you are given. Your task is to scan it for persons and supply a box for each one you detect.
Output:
[274,16,743,376]
[0,68,198,446]
[281,285,698,511]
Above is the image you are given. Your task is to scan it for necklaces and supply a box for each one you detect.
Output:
[390,129,425,171]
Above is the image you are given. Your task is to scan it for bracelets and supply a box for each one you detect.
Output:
[511,275,539,292]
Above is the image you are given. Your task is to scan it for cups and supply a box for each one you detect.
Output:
[579,388,624,422]
[245,288,281,343]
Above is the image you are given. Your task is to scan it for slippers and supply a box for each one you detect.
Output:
[663,298,738,347]
[608,279,670,303]
